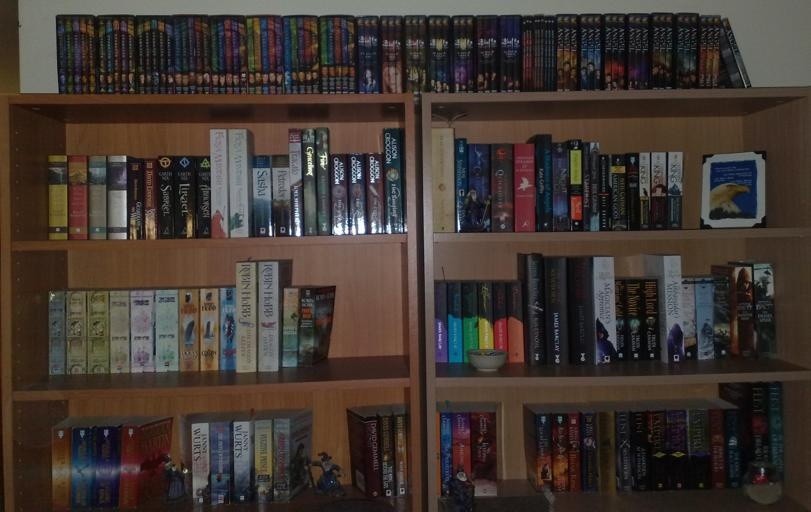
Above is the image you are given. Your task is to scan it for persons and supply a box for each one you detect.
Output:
[164,454,185,499]
[305,451,347,497]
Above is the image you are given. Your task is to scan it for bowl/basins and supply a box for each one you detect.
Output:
[466,348,508,372]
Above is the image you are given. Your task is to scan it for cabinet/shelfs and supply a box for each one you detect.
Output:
[1,93,423,512]
[421,87,811,512]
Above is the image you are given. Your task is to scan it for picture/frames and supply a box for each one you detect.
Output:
[700,150,766,229]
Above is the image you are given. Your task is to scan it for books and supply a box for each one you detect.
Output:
[52,403,173,512]
[523,382,785,493]
[57,14,720,94]
[346,405,406,499]
[436,253,775,364]
[440,401,502,496]
[721,17,752,88]
[179,409,313,502]
[49,128,406,241]
[48,258,337,375]
[432,127,684,233]
